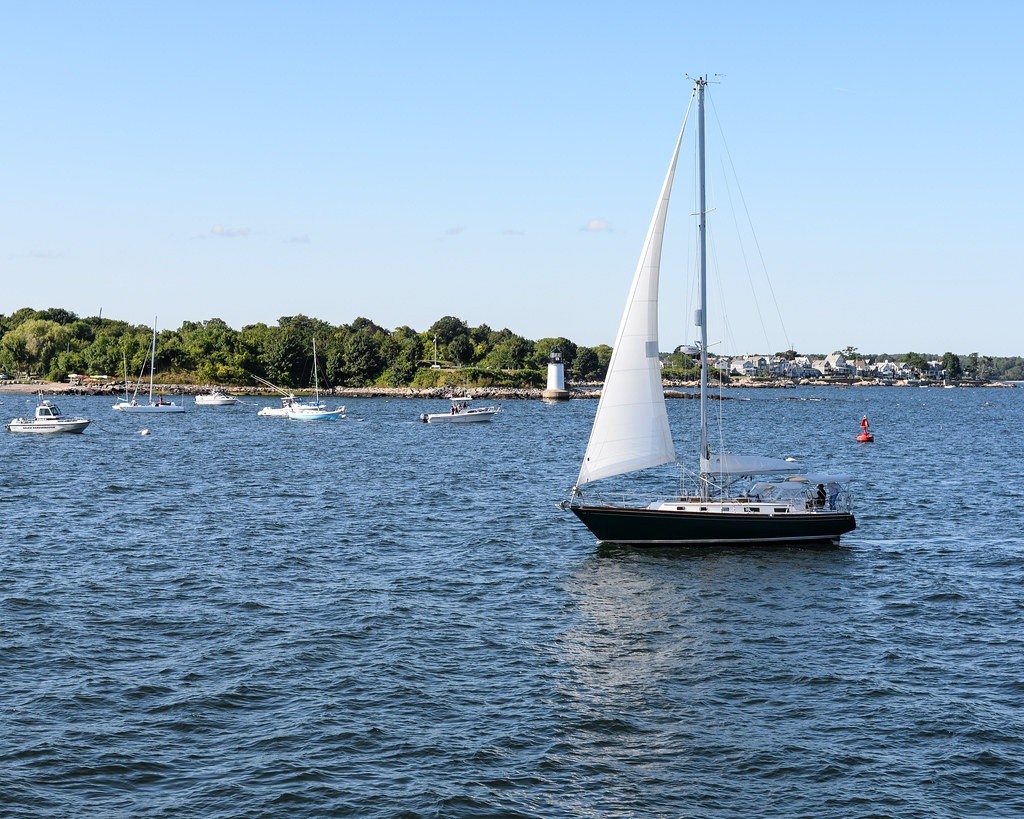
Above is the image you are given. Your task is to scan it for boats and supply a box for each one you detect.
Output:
[7,400,91,433]
[427,396,496,424]
[193,388,237,405]
[258,393,326,416]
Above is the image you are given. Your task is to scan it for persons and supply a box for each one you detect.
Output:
[451,406,454,415]
[828,483,841,510]
[816,484,826,507]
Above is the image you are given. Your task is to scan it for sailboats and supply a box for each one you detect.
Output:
[288,338,345,420]
[119,315,184,412]
[554,72,857,544]
